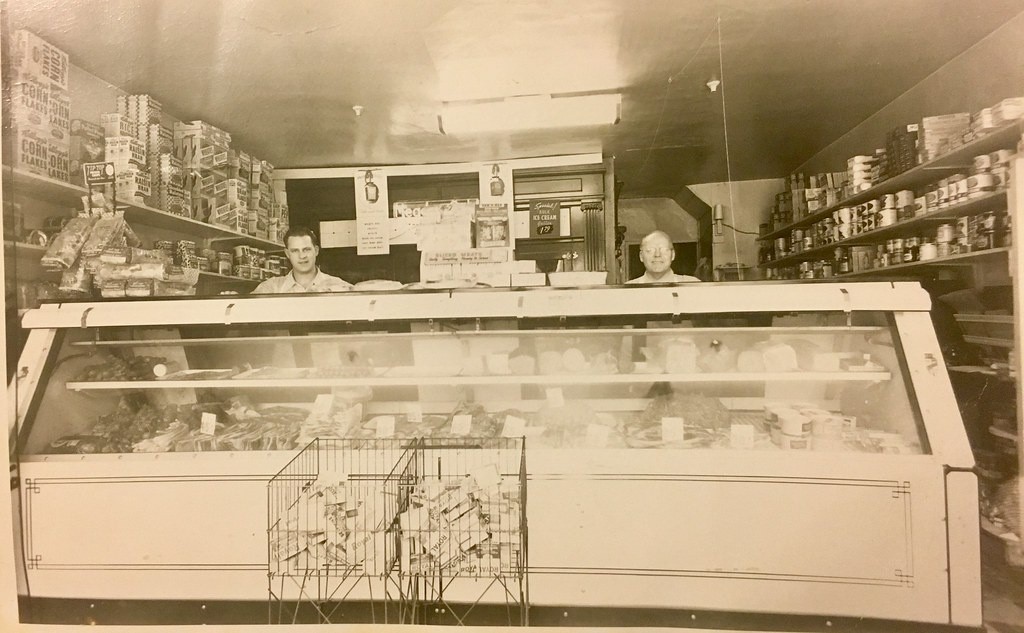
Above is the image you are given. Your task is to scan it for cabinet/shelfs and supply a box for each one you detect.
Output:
[0,164,290,292]
[752,118,1024,280]
[0,279,982,633]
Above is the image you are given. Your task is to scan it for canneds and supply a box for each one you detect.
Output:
[767,148,1018,278]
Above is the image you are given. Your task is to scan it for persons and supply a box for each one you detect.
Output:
[619,230,702,374]
[217,225,356,369]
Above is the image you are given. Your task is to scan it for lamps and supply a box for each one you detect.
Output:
[434,89,625,137]
[489,169,505,196]
[362,171,381,204]
[714,203,726,236]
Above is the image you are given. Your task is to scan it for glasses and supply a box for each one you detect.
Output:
[642,247,674,255]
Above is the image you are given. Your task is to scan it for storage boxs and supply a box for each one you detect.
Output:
[8,28,290,303]
[758,96,1024,273]
